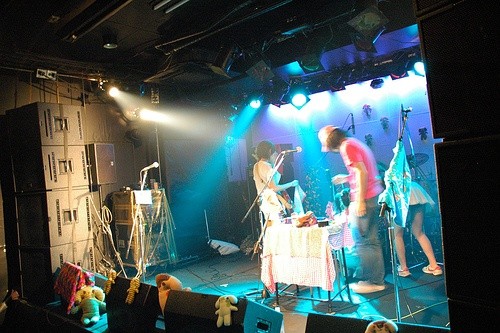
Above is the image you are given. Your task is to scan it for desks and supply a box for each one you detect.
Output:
[260,218,351,314]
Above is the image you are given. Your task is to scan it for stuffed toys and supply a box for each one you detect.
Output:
[215,295,239,328]
[71,263,117,326]
[155,273,191,315]
[362,104,429,148]
[324,201,335,220]
[364,319,398,333]
[125,277,141,305]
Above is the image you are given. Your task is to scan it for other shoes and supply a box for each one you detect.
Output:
[353,280,386,294]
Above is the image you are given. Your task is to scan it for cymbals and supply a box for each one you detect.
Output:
[376,160,388,183]
[398,153,432,171]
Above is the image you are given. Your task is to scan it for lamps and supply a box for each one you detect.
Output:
[98,5,425,124]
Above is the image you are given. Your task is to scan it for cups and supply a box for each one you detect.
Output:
[150,178,156,189]
[153,182,158,189]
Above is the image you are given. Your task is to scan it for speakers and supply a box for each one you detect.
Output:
[449,302,500,333]
[415,1,499,137]
[6,101,84,146]
[434,142,500,302]
[54,262,109,309]
[226,181,253,238]
[15,189,92,247]
[102,277,163,332]
[19,240,97,304]
[163,289,283,333]
[304,312,450,333]
[84,143,118,185]
[9,147,91,192]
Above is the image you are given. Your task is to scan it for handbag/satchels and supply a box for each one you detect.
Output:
[239,234,259,255]
[207,238,241,257]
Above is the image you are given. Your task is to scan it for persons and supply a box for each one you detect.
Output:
[317,124,386,294]
[376,174,443,277]
[252,140,300,221]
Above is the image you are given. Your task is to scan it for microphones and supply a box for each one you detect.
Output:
[402,106,413,113]
[350,114,356,135]
[279,147,302,154]
[140,162,160,171]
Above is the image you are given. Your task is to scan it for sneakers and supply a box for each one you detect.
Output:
[422,264,442,276]
[391,264,410,277]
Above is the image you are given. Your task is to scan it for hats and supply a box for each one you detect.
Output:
[318,125,339,153]
